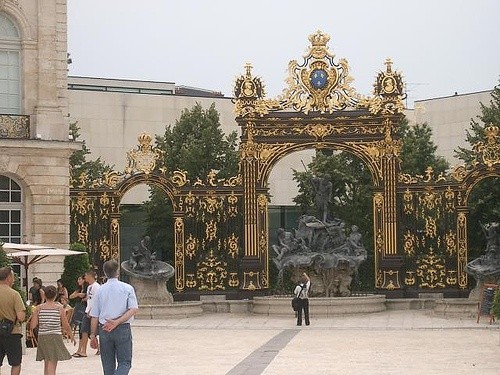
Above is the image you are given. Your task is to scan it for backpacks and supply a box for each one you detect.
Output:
[298,284,308,300]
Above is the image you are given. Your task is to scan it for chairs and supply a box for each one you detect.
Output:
[62,306,76,343]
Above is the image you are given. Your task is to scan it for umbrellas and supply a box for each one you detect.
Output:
[7,248,87,301]
[3,242,56,253]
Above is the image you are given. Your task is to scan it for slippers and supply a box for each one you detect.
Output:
[73,353,87,357]
[96,349,100,355]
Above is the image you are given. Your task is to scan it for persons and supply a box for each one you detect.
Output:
[294,273,311,326]
[30,285,75,375]
[28,277,72,309]
[89,260,138,375]
[485,229,498,252]
[130,235,156,271]
[272,228,294,255]
[69,269,102,357]
[349,225,361,243]
[0,267,26,374]
[312,174,332,223]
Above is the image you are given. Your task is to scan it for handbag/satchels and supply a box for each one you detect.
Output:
[72,302,87,324]
[292,298,302,311]
[0,318,14,336]
